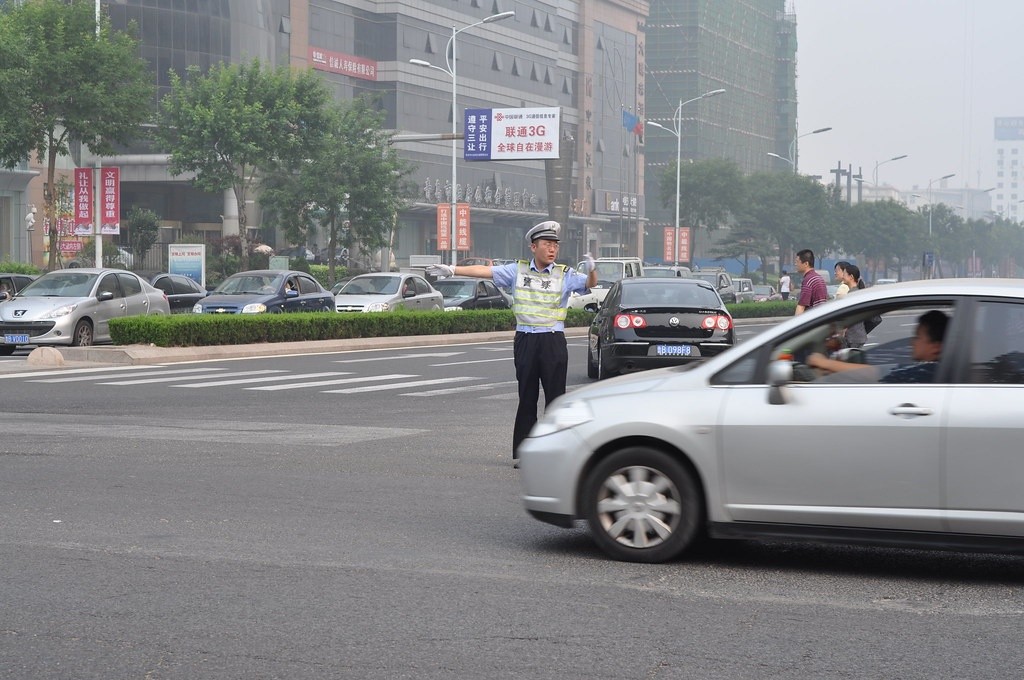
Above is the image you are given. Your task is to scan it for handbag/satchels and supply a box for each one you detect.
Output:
[863,314,882,334]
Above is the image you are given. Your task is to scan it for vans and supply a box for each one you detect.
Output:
[577,255,643,282]
[875,278,898,286]
[456,256,513,266]
[815,270,831,284]
[732,279,753,303]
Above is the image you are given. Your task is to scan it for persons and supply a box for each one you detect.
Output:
[833,260,867,348]
[386,277,407,296]
[612,265,628,277]
[780,270,790,300]
[461,285,481,296]
[312,243,320,265]
[794,248,827,318]
[263,280,292,296]
[694,262,700,269]
[807,311,951,389]
[0,286,10,301]
[424,220,598,468]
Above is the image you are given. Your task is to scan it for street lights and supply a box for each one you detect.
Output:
[767,127,832,272]
[855,154,907,202]
[646,88,727,266]
[410,12,516,268]
[911,173,995,278]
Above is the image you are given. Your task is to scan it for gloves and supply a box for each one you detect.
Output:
[425,264,455,279]
[583,252,595,272]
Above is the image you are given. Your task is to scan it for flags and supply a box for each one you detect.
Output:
[623,110,643,136]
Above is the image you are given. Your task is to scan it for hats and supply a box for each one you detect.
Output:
[525,221,561,244]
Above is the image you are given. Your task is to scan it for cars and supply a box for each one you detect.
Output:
[0,269,170,356]
[278,245,349,265]
[567,287,599,313]
[515,277,1024,564]
[330,273,444,314]
[431,278,514,313]
[133,271,334,314]
[753,284,779,302]
[583,277,735,381]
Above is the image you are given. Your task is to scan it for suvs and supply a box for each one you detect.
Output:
[643,266,734,304]
